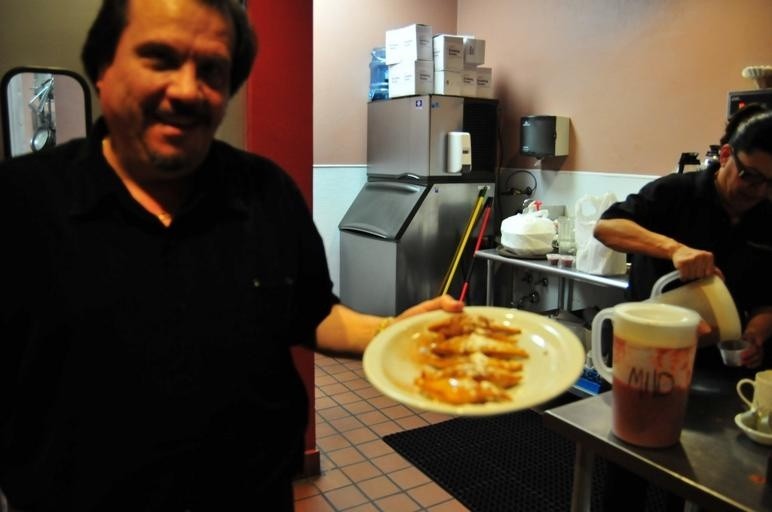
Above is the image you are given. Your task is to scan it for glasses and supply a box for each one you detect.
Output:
[727,142,772,188]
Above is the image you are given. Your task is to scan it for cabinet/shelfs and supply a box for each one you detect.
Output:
[473,244,631,401]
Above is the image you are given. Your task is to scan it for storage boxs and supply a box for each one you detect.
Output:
[382,21,495,101]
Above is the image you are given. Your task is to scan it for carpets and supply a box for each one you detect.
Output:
[378,390,684,510]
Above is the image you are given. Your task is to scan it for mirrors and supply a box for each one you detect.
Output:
[1,63,93,160]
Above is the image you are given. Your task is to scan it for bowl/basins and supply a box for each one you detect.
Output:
[746,65,772,88]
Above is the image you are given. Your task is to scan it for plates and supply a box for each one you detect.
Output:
[734,411,772,445]
[363,305,586,418]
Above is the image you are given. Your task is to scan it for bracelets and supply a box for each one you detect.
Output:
[379,315,394,332]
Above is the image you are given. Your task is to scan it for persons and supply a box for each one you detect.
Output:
[592,112,772,395]
[0,0,465,512]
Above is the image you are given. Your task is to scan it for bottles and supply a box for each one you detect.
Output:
[556,216,576,264]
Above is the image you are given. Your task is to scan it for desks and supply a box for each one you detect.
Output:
[541,390,772,512]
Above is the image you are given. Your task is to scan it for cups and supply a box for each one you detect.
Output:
[717,340,750,367]
[548,252,573,267]
[755,411,772,434]
[736,370,772,416]
